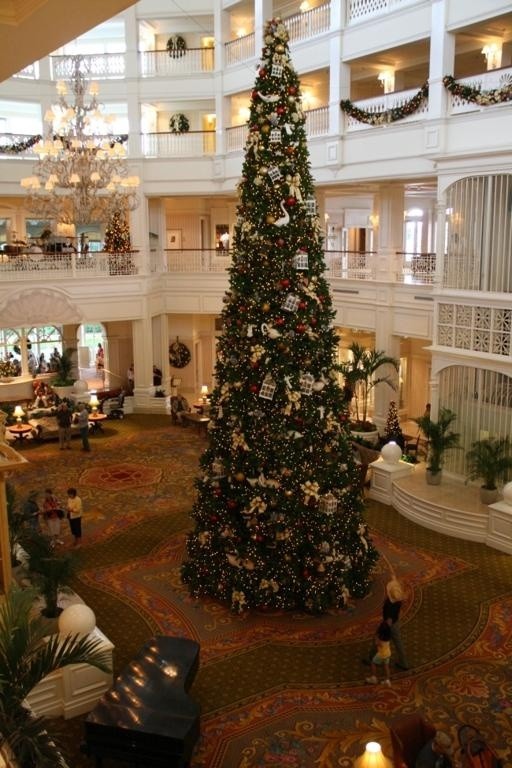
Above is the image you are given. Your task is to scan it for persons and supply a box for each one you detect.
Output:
[127,363,134,395]
[172,393,190,428]
[39,488,65,548]
[1,347,62,376]
[25,502,42,535]
[414,730,453,768]
[56,404,73,450]
[62,488,83,550]
[153,364,163,396]
[96,342,104,374]
[118,385,126,408]
[361,580,410,671]
[423,404,430,444]
[365,621,392,685]
[23,243,43,261]
[74,404,90,452]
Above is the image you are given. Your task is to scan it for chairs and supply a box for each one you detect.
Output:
[170,396,191,425]
[407,434,420,454]
[390,714,436,768]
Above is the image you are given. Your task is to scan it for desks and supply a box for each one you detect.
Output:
[403,435,412,448]
[10,424,33,446]
[88,413,107,435]
[183,413,210,439]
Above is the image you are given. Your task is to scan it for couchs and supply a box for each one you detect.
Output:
[29,413,82,444]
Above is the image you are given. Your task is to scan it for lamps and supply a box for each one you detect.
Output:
[12,406,26,429]
[89,395,101,418]
[20,58,140,226]
[200,385,209,404]
[353,741,394,768]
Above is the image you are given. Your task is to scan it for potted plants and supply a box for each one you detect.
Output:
[23,534,80,636]
[465,435,512,504]
[5,480,37,575]
[331,341,399,447]
[48,347,78,399]
[411,407,463,485]
